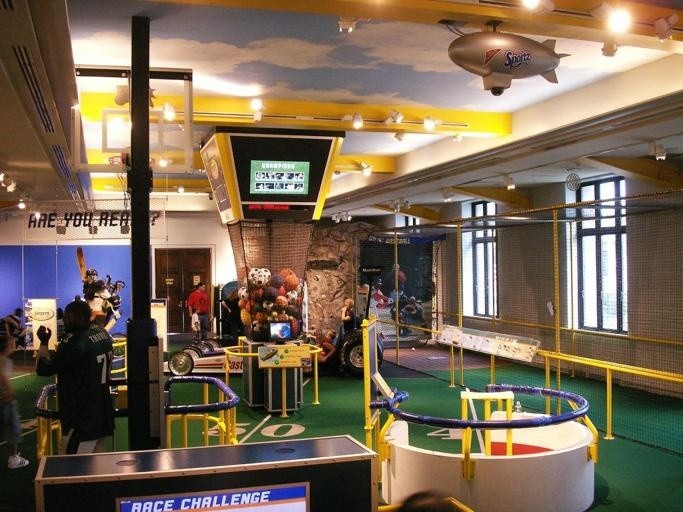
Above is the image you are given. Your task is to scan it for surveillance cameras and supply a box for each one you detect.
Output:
[484,72,513,96]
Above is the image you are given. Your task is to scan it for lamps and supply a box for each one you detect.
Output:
[0,172,130,234]
[352,110,435,129]
[335,174,516,223]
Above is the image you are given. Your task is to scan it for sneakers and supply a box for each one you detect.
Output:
[8,455,30,469]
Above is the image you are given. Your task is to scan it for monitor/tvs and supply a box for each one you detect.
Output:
[268,320,293,344]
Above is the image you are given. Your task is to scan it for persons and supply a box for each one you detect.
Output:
[188,283,211,340]
[342,299,356,338]
[190,308,201,343]
[371,265,431,340]
[313,328,336,355]
[0,295,114,470]
